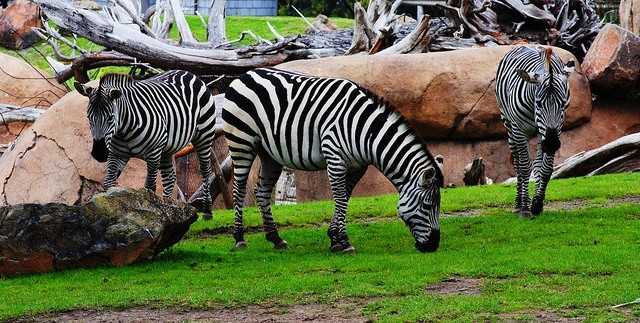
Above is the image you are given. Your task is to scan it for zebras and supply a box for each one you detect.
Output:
[493,42,577,221]
[69,68,218,224]
[220,64,447,255]
